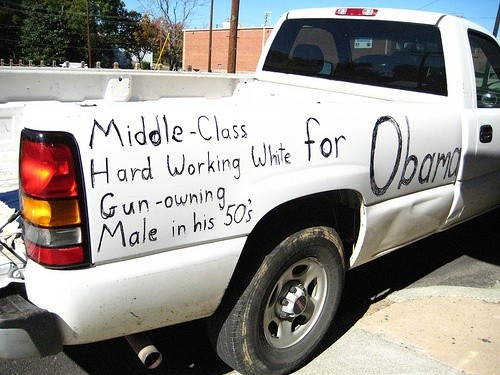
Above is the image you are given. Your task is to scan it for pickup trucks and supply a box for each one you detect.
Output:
[1,5,500,375]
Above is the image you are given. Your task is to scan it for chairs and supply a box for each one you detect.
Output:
[284,43,324,77]
